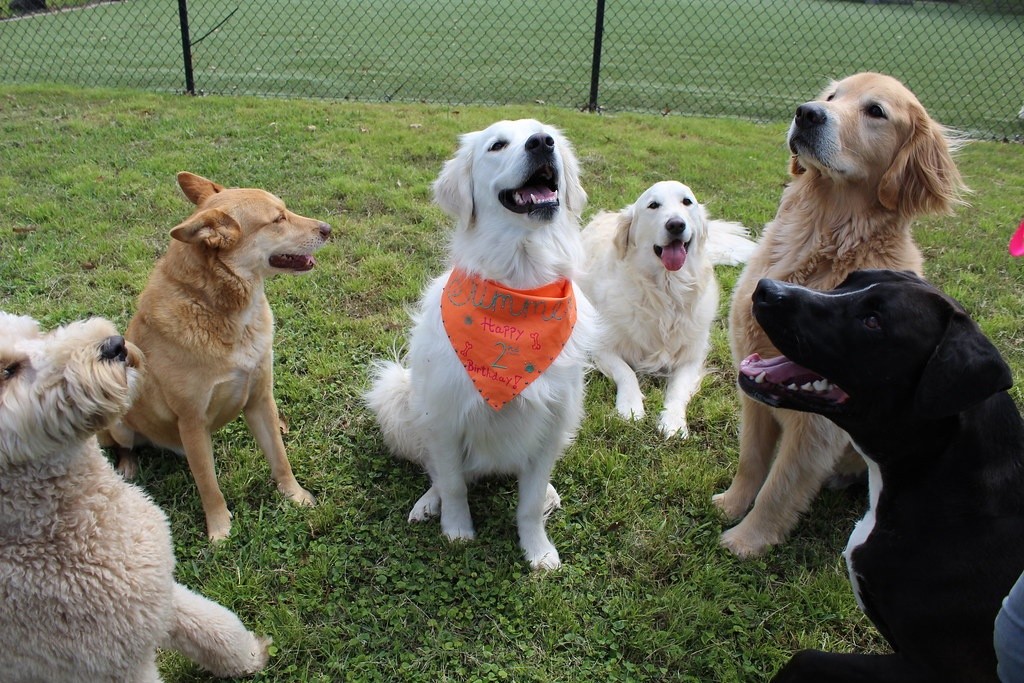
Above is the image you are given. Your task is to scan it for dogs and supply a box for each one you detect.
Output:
[737,268,1024,683]
[710,72,977,562]
[99,170,333,545]
[582,180,756,442]
[0,308,273,683]
[363,117,614,575]
